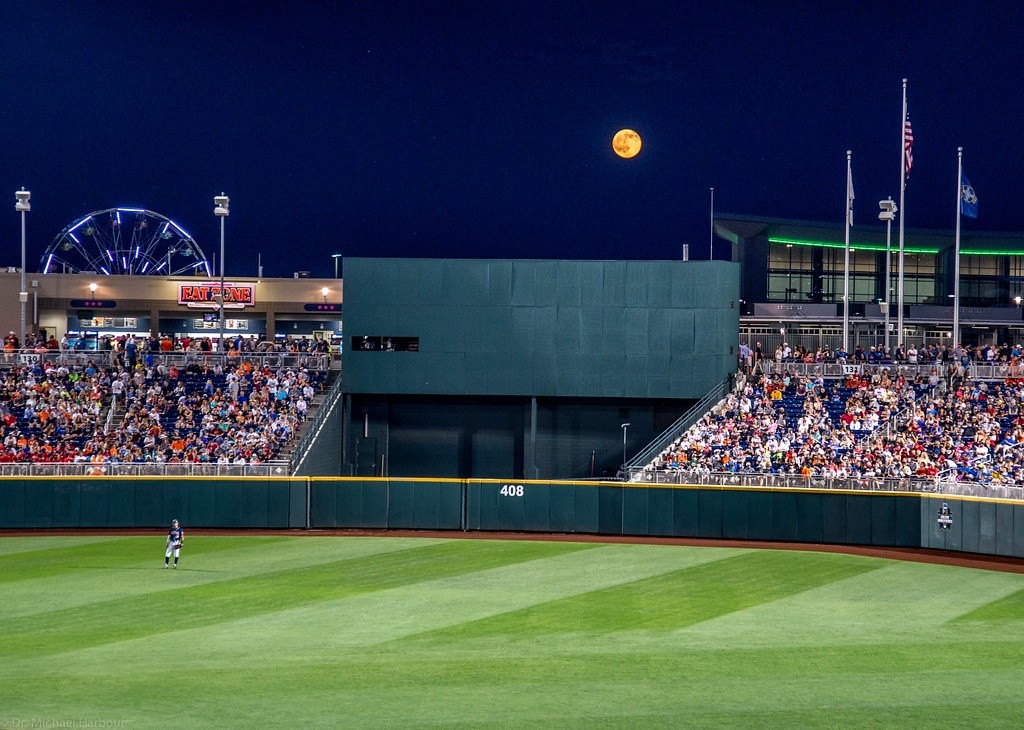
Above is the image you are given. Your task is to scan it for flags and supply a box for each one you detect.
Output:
[848,167,856,226]
[960,168,978,219]
[904,100,914,181]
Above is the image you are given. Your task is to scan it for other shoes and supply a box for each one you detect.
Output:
[163,564,168,569]
[173,564,177,569]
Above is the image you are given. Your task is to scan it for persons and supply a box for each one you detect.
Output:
[164,519,185,569]
[1,329,333,475]
[646,338,1024,495]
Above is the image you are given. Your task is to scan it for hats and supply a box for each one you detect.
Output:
[0,327,333,474]
[647,340,1023,488]
[172,519,178,524]
